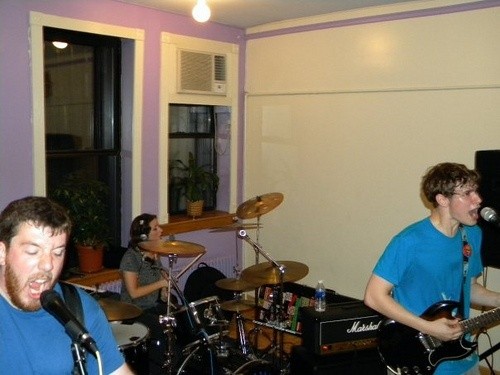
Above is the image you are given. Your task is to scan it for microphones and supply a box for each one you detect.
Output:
[142,255,155,266]
[481,207,500,229]
[40,290,100,354]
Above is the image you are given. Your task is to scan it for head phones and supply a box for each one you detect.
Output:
[140,214,148,241]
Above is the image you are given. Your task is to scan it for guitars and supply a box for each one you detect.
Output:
[375,299,500,375]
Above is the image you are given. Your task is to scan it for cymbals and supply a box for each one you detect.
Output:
[219,299,258,312]
[206,223,263,235]
[213,278,257,292]
[240,261,308,285]
[234,192,283,219]
[94,298,145,321]
[137,240,207,258]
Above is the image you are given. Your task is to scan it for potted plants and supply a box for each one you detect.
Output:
[55,173,116,273]
[172,152,220,217]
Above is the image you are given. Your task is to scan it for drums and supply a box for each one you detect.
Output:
[175,338,275,375]
[162,294,229,353]
[109,321,150,370]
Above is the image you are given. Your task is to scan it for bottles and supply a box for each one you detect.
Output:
[314,280,326,312]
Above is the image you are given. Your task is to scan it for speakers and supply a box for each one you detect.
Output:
[298,300,388,375]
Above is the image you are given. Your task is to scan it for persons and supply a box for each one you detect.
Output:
[120,213,177,318]
[364,163,500,375]
[0,195,136,375]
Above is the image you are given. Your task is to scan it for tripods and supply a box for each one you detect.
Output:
[246,213,288,362]
